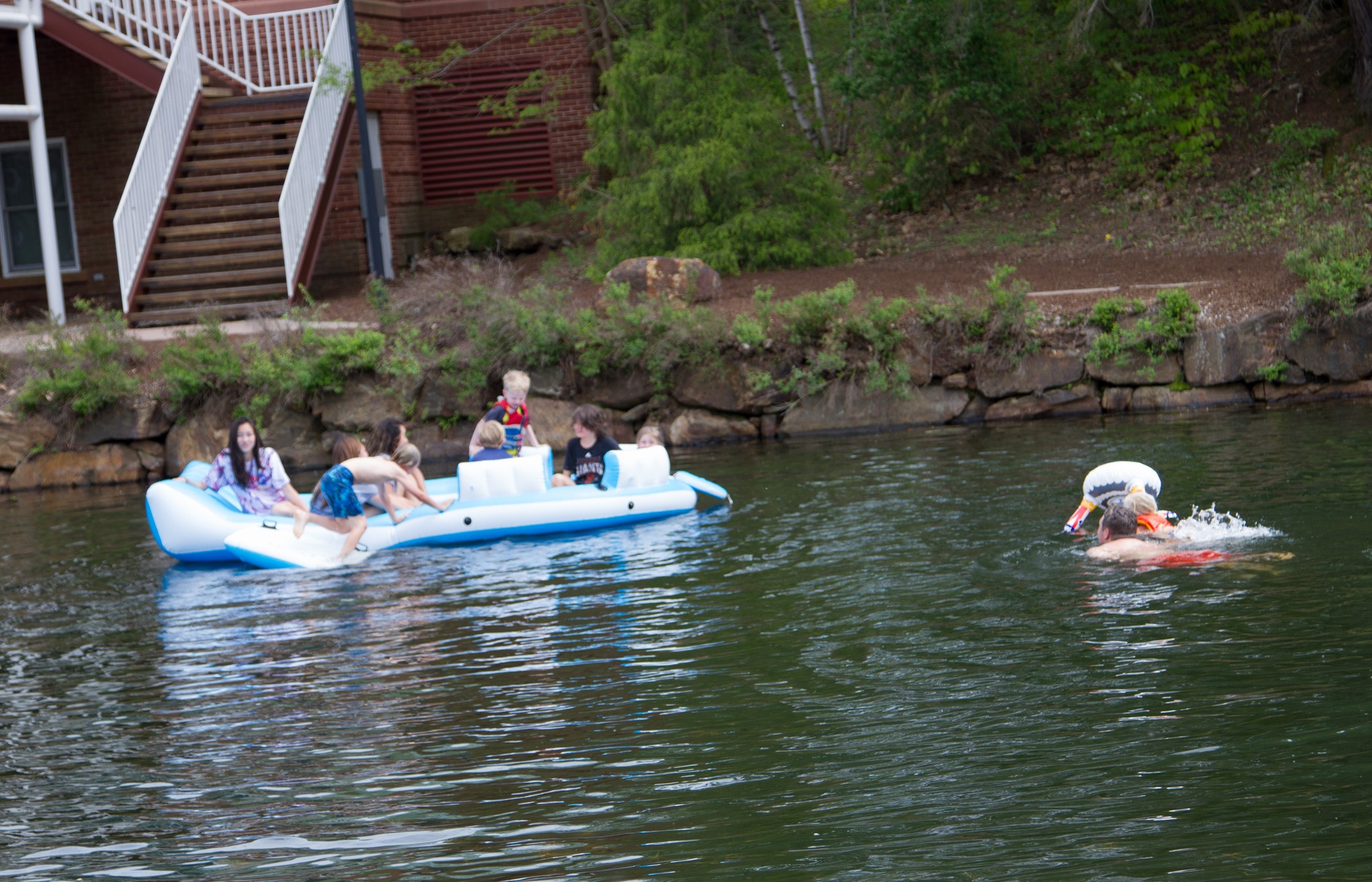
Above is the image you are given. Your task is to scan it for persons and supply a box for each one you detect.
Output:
[637,426,665,448]
[469,370,551,462]
[550,405,622,488]
[173,417,311,515]
[1124,492,1175,534]
[294,441,456,562]
[1086,507,1148,559]
[470,420,514,461]
[332,418,427,520]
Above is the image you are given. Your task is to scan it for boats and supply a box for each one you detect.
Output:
[142,441,729,574]
